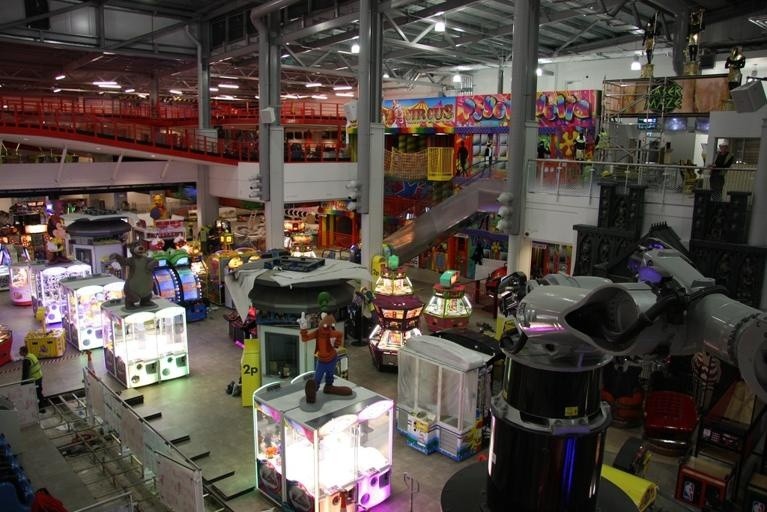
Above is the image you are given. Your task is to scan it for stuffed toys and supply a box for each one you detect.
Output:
[296,292,352,404]
[46,211,70,265]
[107,241,159,310]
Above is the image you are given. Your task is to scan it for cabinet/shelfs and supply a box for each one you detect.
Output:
[131,225,186,251]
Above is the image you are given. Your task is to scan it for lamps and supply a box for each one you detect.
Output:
[453,71,460,82]
[435,16,445,31]
[630,54,641,70]
[350,40,359,53]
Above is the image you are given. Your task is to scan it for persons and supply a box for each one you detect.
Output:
[481,143,492,176]
[458,140,472,176]
[18,346,46,413]
[538,141,549,158]
[574,132,586,175]
[704,141,734,200]
